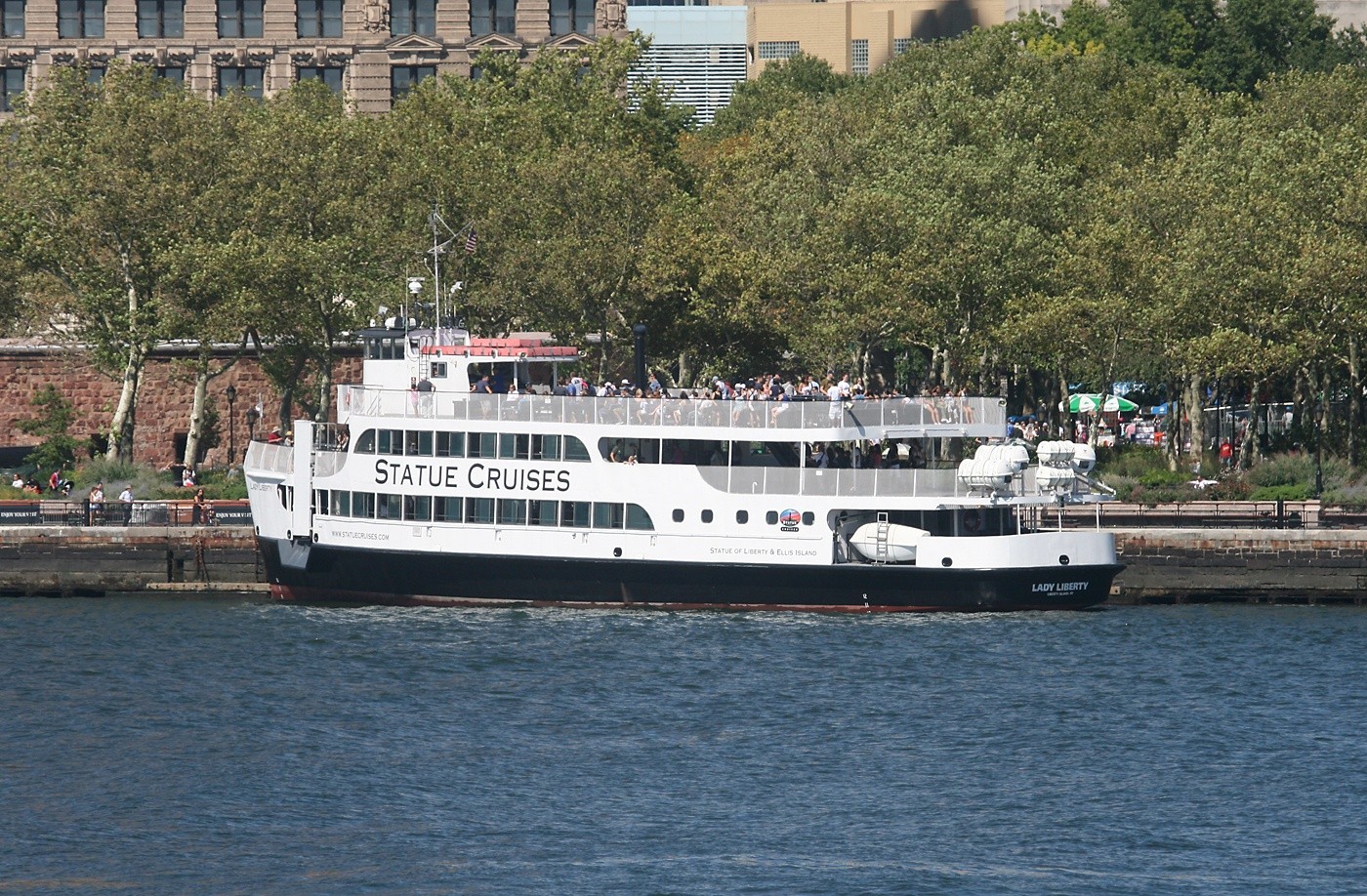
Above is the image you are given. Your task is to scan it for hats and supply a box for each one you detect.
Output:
[622,379,630,385]
[604,382,612,386]
[829,370,835,375]
[286,431,293,436]
[125,485,131,490]
[272,426,281,433]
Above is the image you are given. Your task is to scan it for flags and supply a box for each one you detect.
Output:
[464,225,479,256]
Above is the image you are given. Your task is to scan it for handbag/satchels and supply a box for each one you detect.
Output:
[817,460,821,466]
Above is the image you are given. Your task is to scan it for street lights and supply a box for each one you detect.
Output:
[227,384,236,464]
[1313,404,1324,500]
[245,406,258,441]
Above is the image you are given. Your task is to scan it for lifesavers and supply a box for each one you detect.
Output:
[345,392,358,407]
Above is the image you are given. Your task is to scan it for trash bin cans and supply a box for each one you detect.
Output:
[1301,508,1322,528]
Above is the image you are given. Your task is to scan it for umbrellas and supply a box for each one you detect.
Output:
[1058,392,1138,428]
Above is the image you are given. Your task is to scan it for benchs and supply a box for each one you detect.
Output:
[40,500,84,524]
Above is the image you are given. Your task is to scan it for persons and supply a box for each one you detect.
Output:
[119,484,134,526]
[12,467,106,526]
[283,431,294,447]
[335,430,349,451]
[411,376,435,419]
[469,373,975,427]
[1117,414,1161,443]
[671,442,742,466]
[268,426,283,446]
[1193,475,1205,490]
[1234,417,1247,448]
[433,365,438,376]
[1281,405,1294,431]
[182,464,240,528]
[365,435,375,450]
[1008,416,1090,443]
[811,437,926,469]
[1219,436,1233,470]
[608,439,641,464]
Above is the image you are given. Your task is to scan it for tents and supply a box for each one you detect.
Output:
[1139,386,1244,415]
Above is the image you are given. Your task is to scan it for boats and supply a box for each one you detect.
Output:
[242,212,1129,613]
